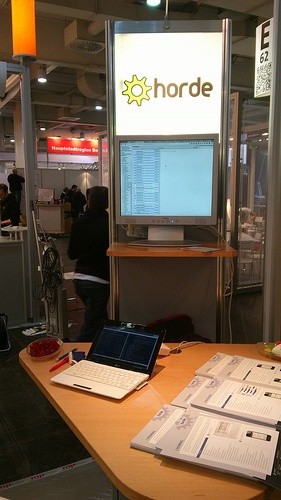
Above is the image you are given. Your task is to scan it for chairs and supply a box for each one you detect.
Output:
[236,240,255,279]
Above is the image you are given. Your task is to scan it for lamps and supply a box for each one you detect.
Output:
[11,0,36,61]
[35,65,47,83]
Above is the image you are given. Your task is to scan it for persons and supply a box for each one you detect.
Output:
[7,169,25,202]
[59,185,87,214]
[66,186,111,340]
[0,183,21,237]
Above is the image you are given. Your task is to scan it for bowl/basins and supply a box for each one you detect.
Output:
[26,337,63,361]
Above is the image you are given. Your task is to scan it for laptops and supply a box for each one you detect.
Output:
[50,317,167,401]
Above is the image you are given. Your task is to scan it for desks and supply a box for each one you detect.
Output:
[35,204,65,234]
[0,234,27,327]
[19,341,281,500]
[227,230,263,278]
[105,240,237,343]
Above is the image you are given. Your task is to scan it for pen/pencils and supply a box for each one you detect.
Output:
[49,356,69,371]
[56,347,78,361]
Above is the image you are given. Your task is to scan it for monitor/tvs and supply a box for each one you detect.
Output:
[113,133,220,246]
[36,204,64,235]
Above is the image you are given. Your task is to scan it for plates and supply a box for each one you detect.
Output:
[255,341,281,360]
